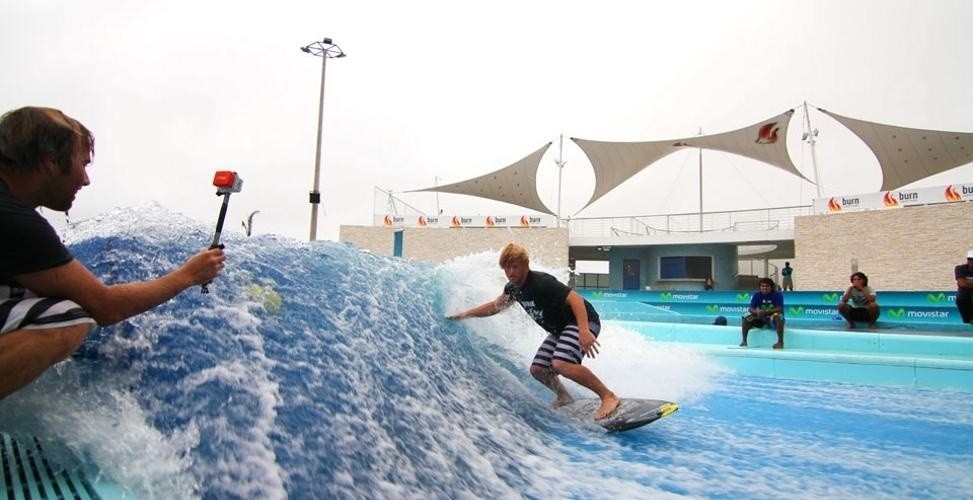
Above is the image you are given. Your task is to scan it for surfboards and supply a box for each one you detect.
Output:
[553,399,678,432]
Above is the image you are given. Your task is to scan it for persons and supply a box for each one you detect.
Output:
[0,106,227,399]
[739,278,785,348]
[954,250,973,324]
[782,261,793,291]
[444,243,620,420]
[839,271,880,330]
